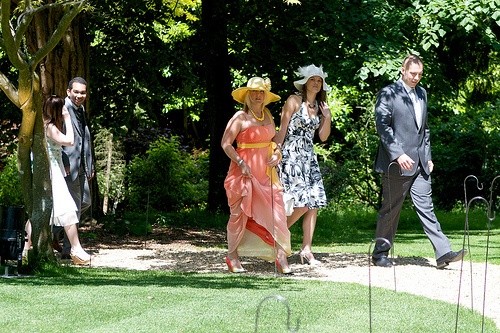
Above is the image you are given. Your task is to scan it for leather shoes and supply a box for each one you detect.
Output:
[436,249,466,269]
[371,255,393,267]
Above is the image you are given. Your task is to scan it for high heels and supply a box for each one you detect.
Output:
[226,256,247,273]
[69,248,98,265]
[299,253,321,265]
[274,257,294,274]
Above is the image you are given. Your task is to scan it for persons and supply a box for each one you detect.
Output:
[272,65,331,265]
[22,76,94,264]
[373,55,468,268]
[221,77,292,274]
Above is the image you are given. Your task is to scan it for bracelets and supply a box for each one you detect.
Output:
[274,150,282,159]
[238,160,243,166]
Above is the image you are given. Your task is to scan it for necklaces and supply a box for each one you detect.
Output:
[250,108,264,121]
[308,104,316,108]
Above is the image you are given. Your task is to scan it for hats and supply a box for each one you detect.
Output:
[293,64,329,92]
[231,76,281,105]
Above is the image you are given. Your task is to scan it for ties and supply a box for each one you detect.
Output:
[409,89,422,129]
[77,108,84,136]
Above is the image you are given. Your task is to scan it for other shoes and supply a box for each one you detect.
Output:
[61,254,71,260]
